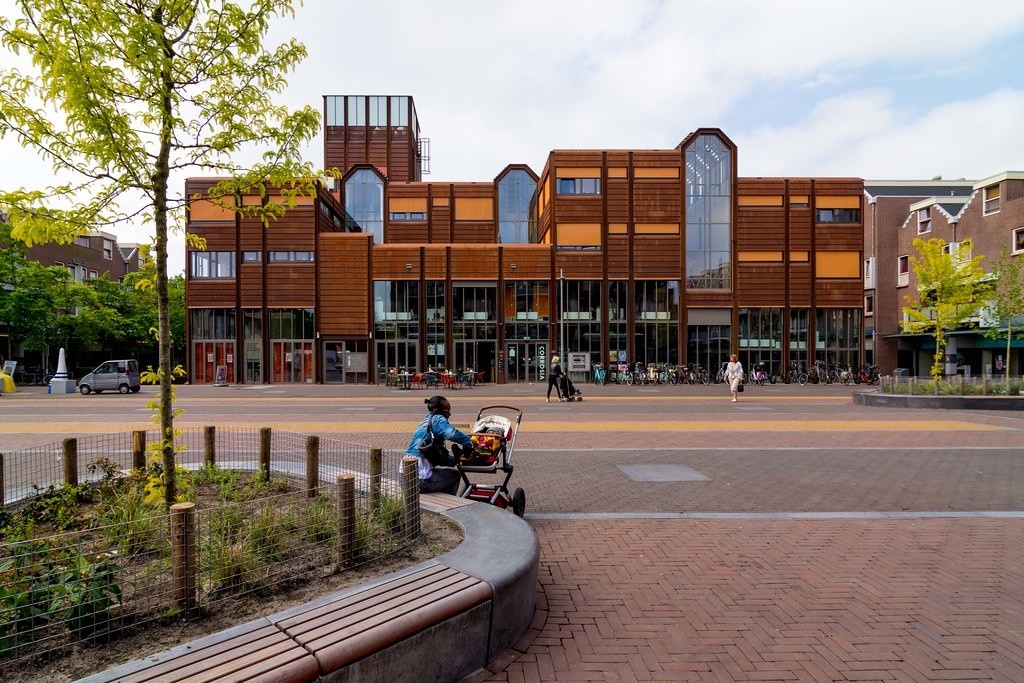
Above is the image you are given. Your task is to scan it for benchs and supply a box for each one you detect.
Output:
[276,563,492,683]
[327,469,477,510]
[4,460,340,506]
[433,503,541,663]
[108,624,321,683]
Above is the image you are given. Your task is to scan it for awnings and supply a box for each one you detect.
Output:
[882,325,1024,337]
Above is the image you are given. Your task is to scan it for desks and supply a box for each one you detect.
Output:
[441,374,457,390]
[397,374,412,390]
[464,371,478,388]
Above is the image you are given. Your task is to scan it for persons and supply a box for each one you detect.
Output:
[399,396,475,497]
[451,427,504,465]
[545,356,567,402]
[724,354,743,402]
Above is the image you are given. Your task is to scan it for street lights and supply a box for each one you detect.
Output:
[559,268,566,374]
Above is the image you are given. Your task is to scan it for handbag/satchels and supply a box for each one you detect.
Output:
[738,381,744,392]
[418,414,449,466]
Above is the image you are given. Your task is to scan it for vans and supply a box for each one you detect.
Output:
[79,359,141,395]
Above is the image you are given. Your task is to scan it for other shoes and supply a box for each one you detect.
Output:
[546,398,548,403]
[464,443,472,458]
[559,398,563,402]
[451,444,462,460]
[731,399,737,402]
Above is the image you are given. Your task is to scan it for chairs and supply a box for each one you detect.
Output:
[376,371,485,390]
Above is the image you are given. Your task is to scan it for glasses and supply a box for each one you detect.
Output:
[444,410,450,413]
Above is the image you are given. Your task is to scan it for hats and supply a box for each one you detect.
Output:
[552,356,559,363]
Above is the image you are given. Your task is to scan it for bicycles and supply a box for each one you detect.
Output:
[783,360,881,386]
[591,361,607,386]
[619,361,777,387]
[16,367,54,386]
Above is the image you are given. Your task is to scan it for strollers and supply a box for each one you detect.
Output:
[558,373,583,402]
[451,405,526,517]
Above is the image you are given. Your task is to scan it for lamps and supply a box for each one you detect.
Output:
[511,263,516,270]
[406,263,412,270]
